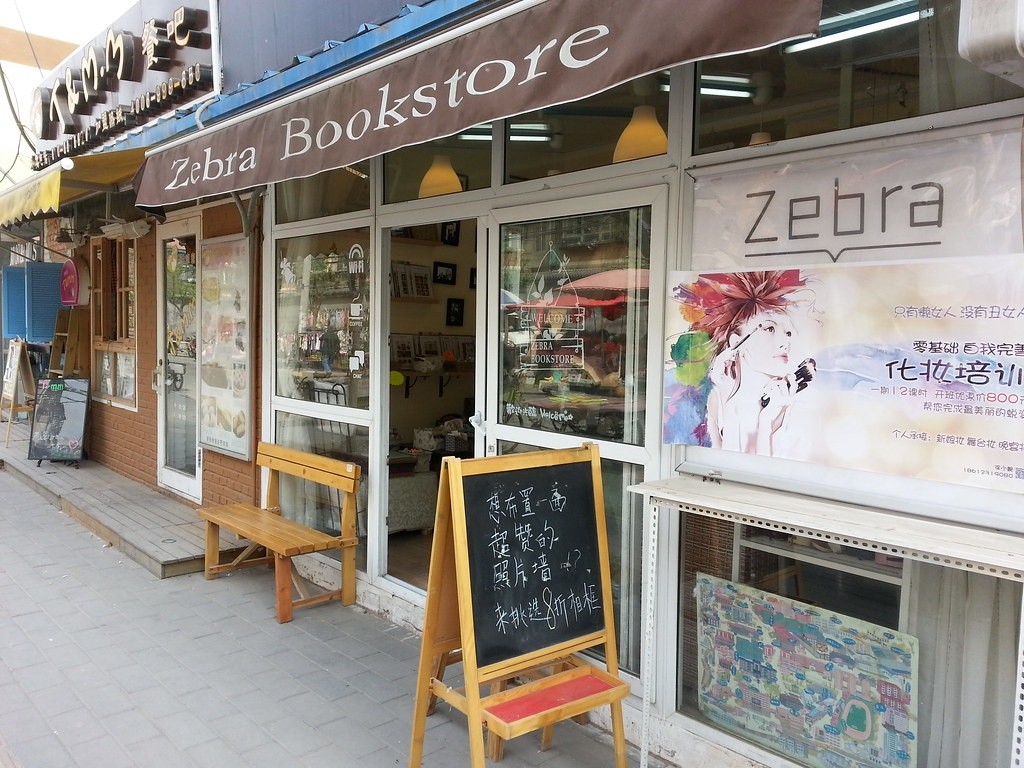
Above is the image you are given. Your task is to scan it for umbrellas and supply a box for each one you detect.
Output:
[553,268,649,377]
[506,290,627,380]
[500,288,525,332]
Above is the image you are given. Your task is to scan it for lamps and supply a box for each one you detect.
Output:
[777,2,935,56]
[56,226,85,244]
[611,104,668,164]
[417,154,462,199]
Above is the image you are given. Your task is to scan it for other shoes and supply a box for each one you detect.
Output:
[326,373,332,378]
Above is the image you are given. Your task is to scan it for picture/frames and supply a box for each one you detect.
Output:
[389,221,477,370]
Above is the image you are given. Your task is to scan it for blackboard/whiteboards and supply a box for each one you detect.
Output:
[449,442,620,683]
[1,339,22,403]
[27,377,91,461]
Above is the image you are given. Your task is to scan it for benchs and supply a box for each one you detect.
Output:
[193,440,362,625]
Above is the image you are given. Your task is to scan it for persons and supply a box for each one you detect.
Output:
[320,322,341,377]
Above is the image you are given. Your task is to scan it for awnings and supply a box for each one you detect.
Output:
[134,0,822,207]
[0,145,151,230]
